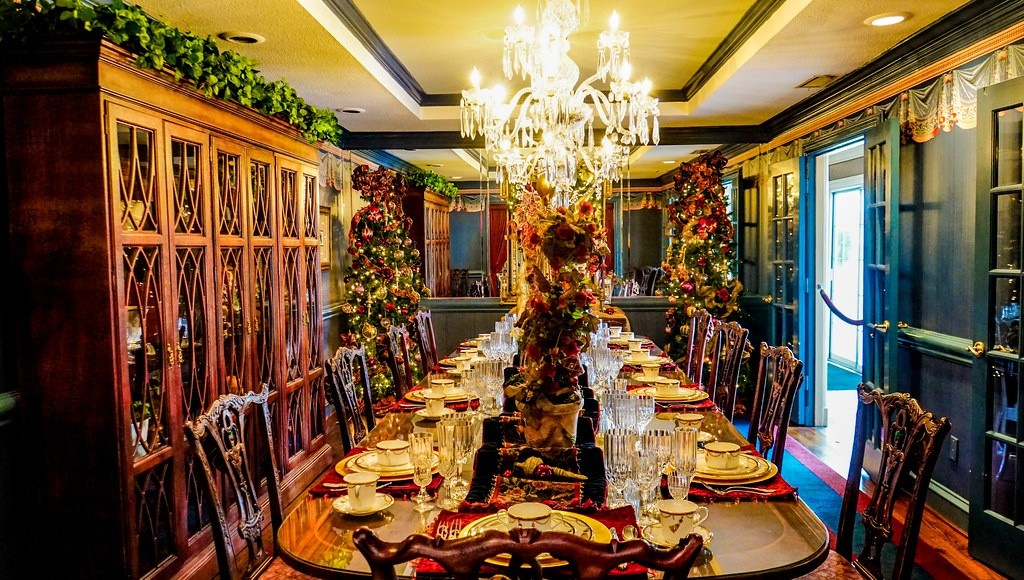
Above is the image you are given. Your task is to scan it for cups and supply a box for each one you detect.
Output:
[343,472,380,511]
[704,441,740,469]
[656,499,709,544]
[631,349,650,361]
[423,394,446,415]
[479,333,491,340]
[430,379,454,396]
[620,332,634,341]
[475,338,488,350]
[627,339,641,350]
[497,502,552,532]
[641,364,660,379]
[610,326,622,336]
[460,349,477,362]
[656,379,681,396]
[675,412,704,436]
[454,357,471,370]
[376,439,409,465]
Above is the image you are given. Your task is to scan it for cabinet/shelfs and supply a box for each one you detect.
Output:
[404,189,456,297]
[1,42,330,579]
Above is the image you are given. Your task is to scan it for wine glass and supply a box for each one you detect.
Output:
[408,310,517,511]
[585,317,697,519]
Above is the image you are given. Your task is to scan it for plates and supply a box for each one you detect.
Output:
[456,511,612,569]
[627,387,709,403]
[696,432,713,442]
[414,408,456,421]
[661,449,778,485]
[333,493,395,517]
[334,450,440,481]
[641,523,713,546]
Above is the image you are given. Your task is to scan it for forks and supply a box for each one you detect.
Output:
[698,480,775,496]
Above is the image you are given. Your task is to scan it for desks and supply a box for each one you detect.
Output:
[279,333,828,580]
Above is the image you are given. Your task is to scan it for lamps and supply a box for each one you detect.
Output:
[461,0,662,206]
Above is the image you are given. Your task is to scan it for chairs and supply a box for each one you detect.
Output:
[324,345,376,454]
[633,265,660,294]
[749,340,804,469]
[683,308,710,385]
[385,322,413,398]
[184,382,331,579]
[354,528,705,580]
[446,265,494,297]
[773,384,953,579]
[413,310,443,375]
[710,318,747,422]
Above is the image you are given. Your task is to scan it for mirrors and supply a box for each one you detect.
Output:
[380,143,763,307]
